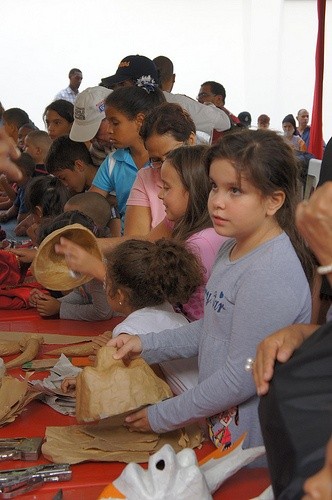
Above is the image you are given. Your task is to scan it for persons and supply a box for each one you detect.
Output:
[302,438,331,499]
[48,142,232,324]
[0,53,332,285]
[103,129,318,473]
[27,211,125,322]
[58,238,201,395]
[255,317,331,499]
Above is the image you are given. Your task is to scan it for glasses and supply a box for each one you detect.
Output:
[196,93,216,100]
[148,158,164,170]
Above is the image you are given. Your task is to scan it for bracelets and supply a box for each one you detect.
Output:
[316,264,332,275]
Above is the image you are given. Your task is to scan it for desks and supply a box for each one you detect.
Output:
[0,309,271,500]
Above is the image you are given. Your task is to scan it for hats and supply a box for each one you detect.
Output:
[68,86,115,143]
[237,112,251,125]
[101,54,159,88]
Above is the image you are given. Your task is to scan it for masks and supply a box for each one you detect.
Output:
[282,128,295,138]
